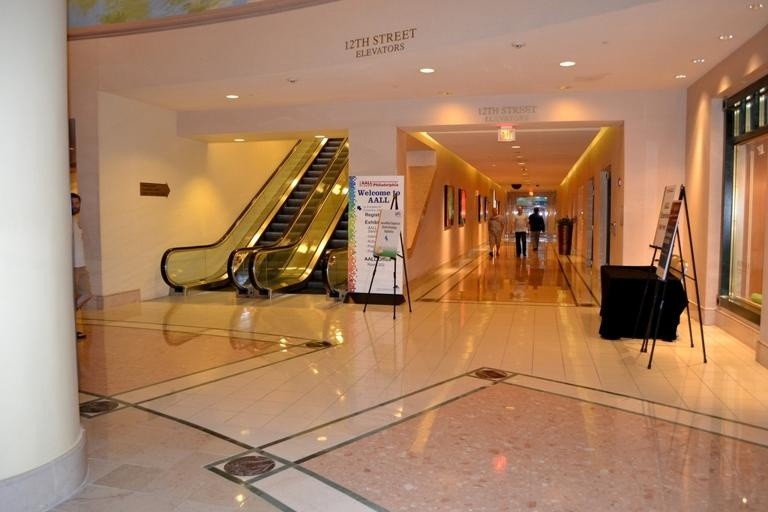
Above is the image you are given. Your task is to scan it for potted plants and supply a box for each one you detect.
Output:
[555,214,573,256]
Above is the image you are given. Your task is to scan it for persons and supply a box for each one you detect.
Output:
[487,258,503,300]
[488,208,504,258]
[511,205,530,256]
[530,250,545,291]
[71,193,92,339]
[528,208,545,250]
[510,256,529,301]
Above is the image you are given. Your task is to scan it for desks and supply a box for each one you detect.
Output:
[593,263,690,342]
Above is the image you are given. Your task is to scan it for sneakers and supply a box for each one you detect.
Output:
[516,253,526,258]
[489,252,494,257]
[77,330,87,338]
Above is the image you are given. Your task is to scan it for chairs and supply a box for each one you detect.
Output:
[668,252,688,283]
